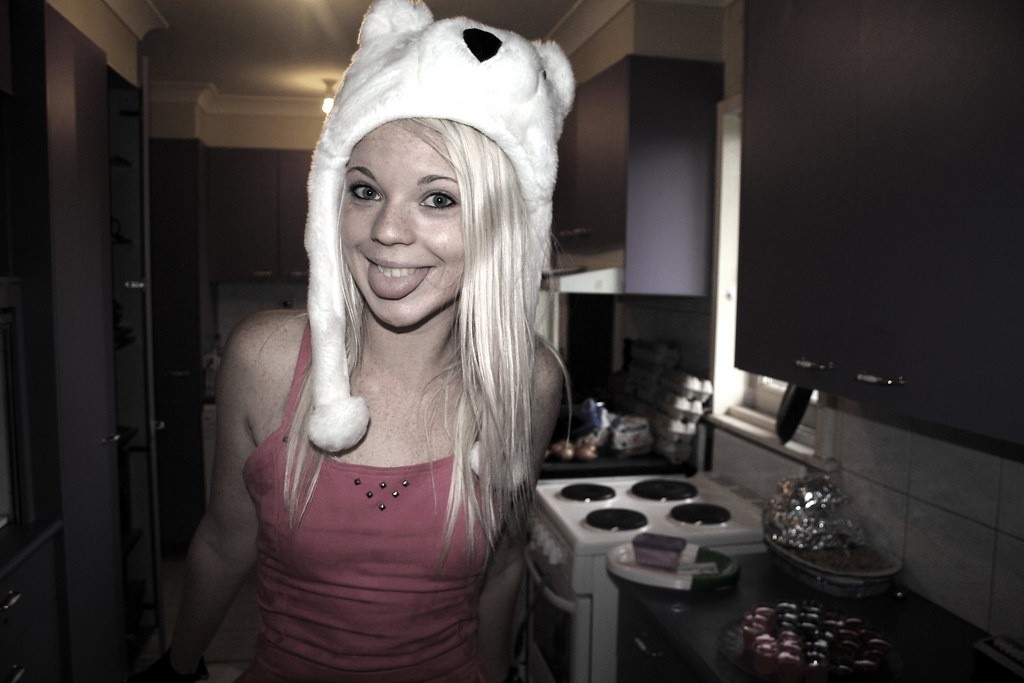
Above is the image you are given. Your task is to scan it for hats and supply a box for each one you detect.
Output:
[301,0,576,491]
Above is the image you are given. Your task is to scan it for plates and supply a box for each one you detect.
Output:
[605,541,738,593]
[718,608,772,681]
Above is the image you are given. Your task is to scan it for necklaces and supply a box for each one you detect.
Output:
[144,0,576,683]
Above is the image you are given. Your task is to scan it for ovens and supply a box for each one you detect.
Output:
[509,549,618,683]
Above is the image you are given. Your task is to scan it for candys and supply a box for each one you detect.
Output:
[742,603,891,682]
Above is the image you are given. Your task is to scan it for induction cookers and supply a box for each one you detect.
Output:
[529,470,765,595]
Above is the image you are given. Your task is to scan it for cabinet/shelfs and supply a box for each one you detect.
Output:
[735,0,1024,445]
[550,54,724,298]
[0,0,312,683]
